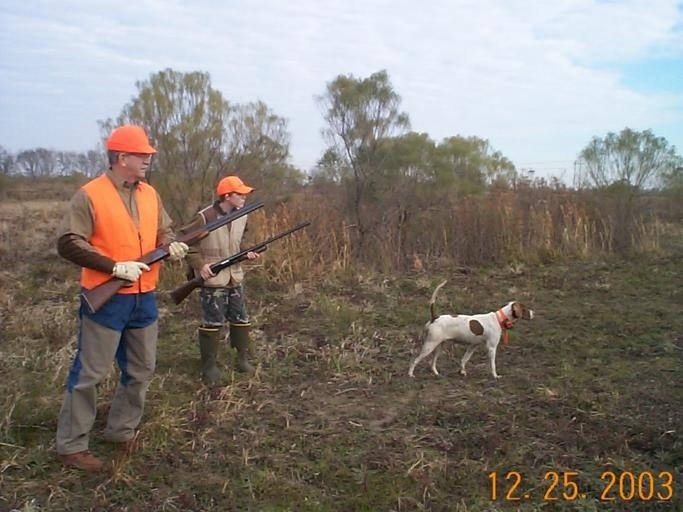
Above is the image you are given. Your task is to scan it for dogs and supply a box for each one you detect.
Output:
[407,278,535,379]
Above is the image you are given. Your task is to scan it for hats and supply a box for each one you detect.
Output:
[106,125,157,154]
[217,175,255,195]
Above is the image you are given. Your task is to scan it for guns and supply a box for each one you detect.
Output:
[79,199,265,316]
[170,222,311,306]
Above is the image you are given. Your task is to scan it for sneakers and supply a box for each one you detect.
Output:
[118,439,142,450]
[63,449,102,470]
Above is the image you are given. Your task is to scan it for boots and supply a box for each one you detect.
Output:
[229,322,255,372]
[198,325,223,380]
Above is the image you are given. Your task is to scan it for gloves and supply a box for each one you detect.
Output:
[111,261,150,282]
[166,241,189,262]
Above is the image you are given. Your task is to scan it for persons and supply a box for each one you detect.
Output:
[56,124,190,471]
[175,175,260,387]
